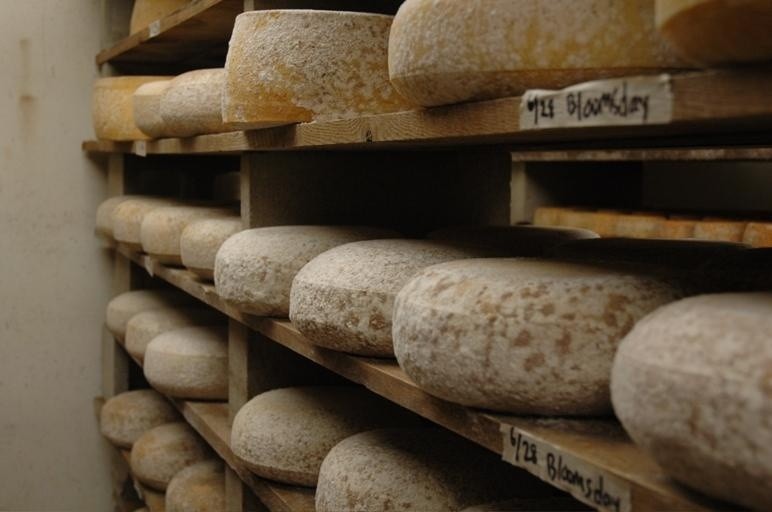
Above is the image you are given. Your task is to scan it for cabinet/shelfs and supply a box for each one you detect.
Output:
[83,1,769,510]
